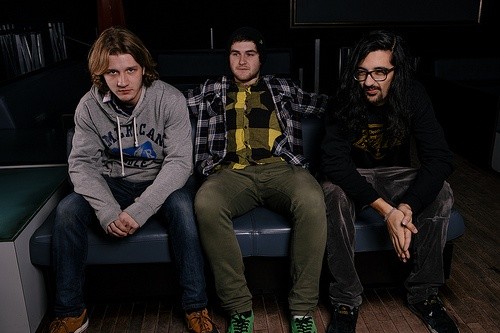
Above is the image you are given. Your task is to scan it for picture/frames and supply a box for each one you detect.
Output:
[289,0,483,30]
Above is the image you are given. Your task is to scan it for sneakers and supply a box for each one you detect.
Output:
[48,308,90,333]
[185,307,221,333]
[290,315,317,333]
[325,305,358,333]
[227,309,255,333]
[408,293,460,333]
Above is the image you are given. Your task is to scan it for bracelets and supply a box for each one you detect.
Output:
[383,206,396,223]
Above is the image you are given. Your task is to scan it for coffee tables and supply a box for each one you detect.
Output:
[0,163,77,333]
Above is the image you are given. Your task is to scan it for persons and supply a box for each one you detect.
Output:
[312,30,462,333]
[178,32,335,333]
[43,28,220,333]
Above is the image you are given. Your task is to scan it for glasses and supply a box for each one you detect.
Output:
[353,66,395,82]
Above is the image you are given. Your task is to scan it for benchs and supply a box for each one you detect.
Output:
[30,119,466,315]
